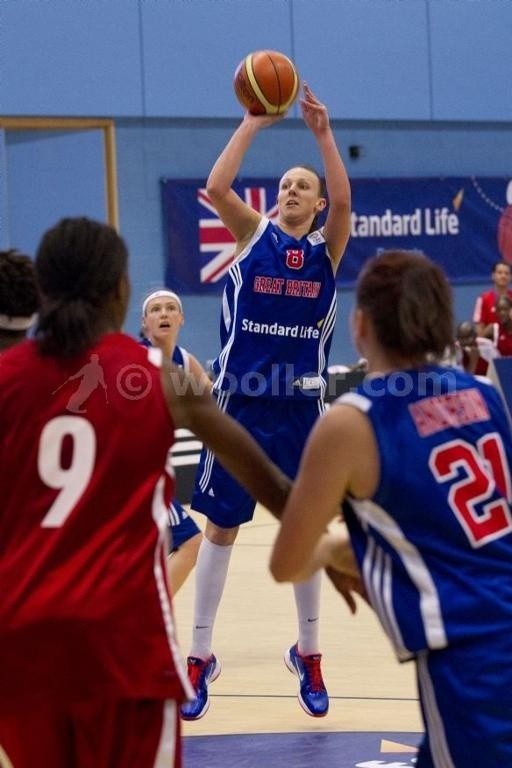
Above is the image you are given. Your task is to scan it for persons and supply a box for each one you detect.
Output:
[451,320,501,382]
[470,259,512,376]
[1,244,46,364]
[474,296,512,360]
[0,211,372,767]
[131,284,218,602]
[267,247,512,767]
[177,81,351,723]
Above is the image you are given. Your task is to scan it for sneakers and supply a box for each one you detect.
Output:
[178,650,223,722]
[283,641,331,720]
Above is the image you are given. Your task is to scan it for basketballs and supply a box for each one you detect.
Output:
[234,50,300,115]
